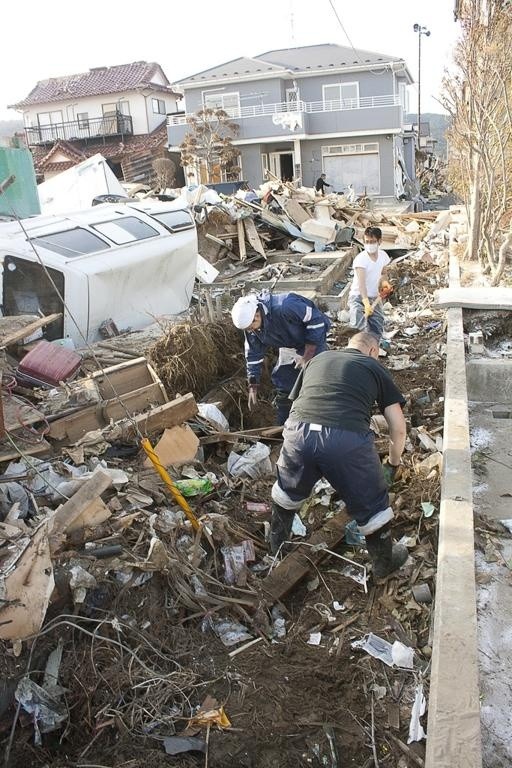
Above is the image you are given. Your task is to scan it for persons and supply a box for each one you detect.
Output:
[268,330,410,586]
[314,172,333,195]
[345,227,394,352]
[230,292,330,438]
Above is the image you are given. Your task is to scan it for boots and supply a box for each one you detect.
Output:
[270,496,301,554]
[366,522,408,586]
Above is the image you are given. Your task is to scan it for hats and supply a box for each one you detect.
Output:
[232,295,259,331]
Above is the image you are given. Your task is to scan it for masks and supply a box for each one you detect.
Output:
[364,244,379,254]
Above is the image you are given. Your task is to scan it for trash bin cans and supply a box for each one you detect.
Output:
[206,180,249,194]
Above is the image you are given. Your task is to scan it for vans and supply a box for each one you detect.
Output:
[0,200,198,349]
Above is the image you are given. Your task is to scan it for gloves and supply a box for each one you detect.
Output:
[381,460,398,489]
[363,298,373,315]
[381,280,393,291]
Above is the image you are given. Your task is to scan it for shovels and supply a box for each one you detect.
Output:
[334,295,379,346]
